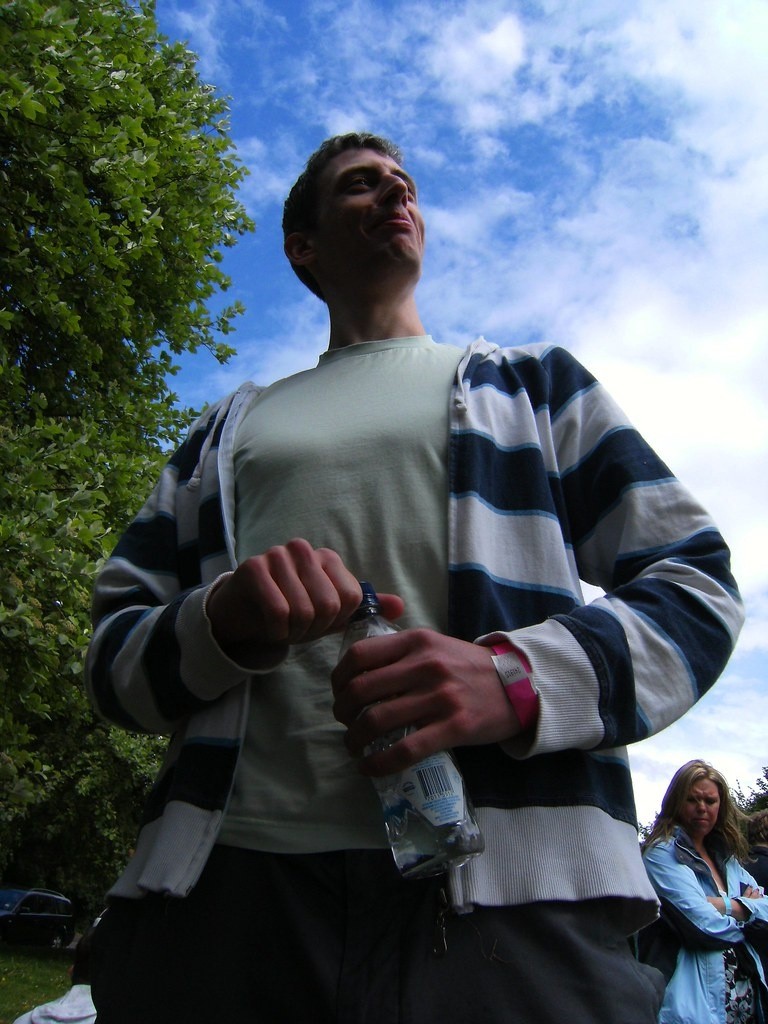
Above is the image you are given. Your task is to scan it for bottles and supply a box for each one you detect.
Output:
[338,581,485,881]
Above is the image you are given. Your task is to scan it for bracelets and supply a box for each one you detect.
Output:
[723,897,731,915]
[492,642,539,727]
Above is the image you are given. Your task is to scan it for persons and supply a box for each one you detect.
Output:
[86,133,744,1024]
[13,926,97,1024]
[641,760,768,1024]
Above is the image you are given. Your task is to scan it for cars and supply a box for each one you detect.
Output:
[0,887,76,950]
